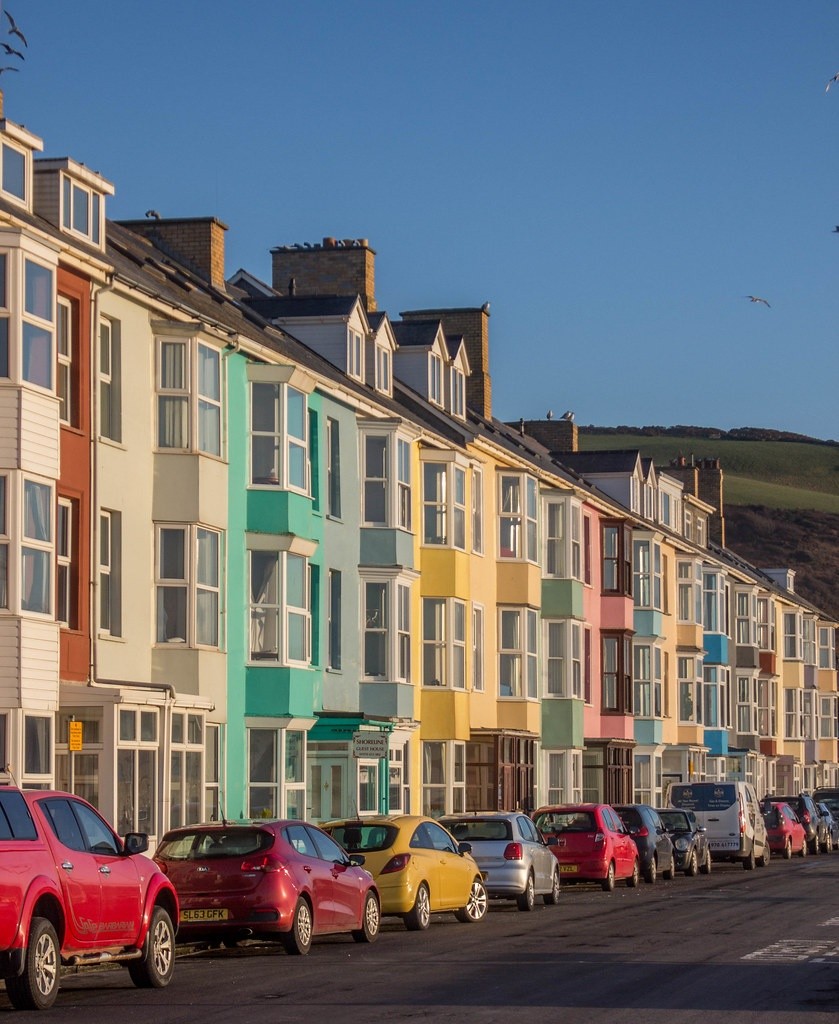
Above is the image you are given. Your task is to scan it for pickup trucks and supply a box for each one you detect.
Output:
[0,786,182,1010]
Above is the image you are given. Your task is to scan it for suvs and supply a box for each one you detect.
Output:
[762,794,828,856]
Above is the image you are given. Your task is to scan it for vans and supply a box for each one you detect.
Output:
[665,780,771,870]
[812,784,839,824]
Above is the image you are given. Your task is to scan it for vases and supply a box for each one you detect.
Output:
[255,476,279,484]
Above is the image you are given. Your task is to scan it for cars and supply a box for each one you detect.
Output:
[757,802,807,859]
[436,810,560,911]
[535,802,641,893]
[815,802,839,853]
[609,802,676,882]
[657,806,712,879]
[319,814,488,930]
[153,818,383,958]
